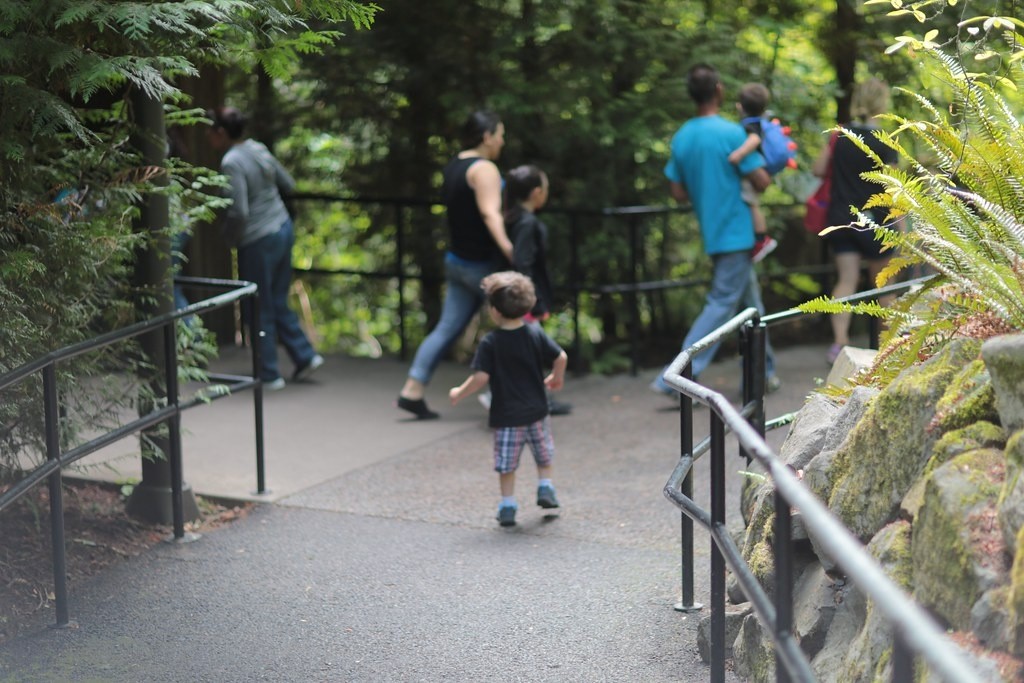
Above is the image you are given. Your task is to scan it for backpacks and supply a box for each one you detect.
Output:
[741,115,799,175]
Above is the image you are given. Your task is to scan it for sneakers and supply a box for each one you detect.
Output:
[750,235,779,263]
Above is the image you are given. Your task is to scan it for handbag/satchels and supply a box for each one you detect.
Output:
[805,124,844,234]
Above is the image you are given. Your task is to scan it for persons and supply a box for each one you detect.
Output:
[652,62,782,407]
[128,132,203,396]
[450,271,567,528]
[203,107,324,390]
[818,78,908,364]
[478,164,575,416]
[397,111,513,419]
[728,81,778,263]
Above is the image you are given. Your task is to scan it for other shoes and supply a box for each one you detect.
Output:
[651,374,700,405]
[292,352,324,381]
[257,370,285,391]
[828,344,841,364]
[547,399,570,415]
[395,395,438,420]
[496,502,516,525]
[765,372,780,392]
[537,486,559,508]
